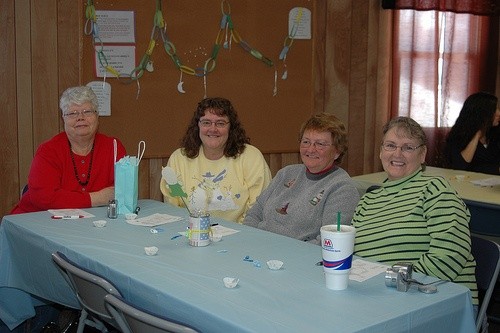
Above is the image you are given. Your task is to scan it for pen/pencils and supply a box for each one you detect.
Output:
[51,215,86,219]
[315,261,323,266]
[188,223,219,229]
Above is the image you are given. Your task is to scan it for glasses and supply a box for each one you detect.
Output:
[64,109,94,118]
[381,142,422,154]
[198,119,230,129]
[300,138,335,151]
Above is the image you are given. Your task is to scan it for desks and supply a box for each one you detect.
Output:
[350,166,500,210]
[0,200,476,333]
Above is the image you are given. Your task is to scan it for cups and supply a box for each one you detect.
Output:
[320,224,356,291]
[188,213,211,246]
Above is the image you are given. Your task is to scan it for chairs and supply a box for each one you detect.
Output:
[51,253,198,333]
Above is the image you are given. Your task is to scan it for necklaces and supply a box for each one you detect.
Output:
[68,139,95,185]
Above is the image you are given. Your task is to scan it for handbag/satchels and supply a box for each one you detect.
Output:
[113,138,145,216]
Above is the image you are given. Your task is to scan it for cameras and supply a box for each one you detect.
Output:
[384,262,414,291]
[107,200,118,218]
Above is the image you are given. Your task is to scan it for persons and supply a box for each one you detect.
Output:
[10,85,127,333]
[243,113,360,245]
[160,98,273,224]
[351,116,479,321]
[440,91,500,320]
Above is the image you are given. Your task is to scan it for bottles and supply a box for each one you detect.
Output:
[107,199,118,219]
